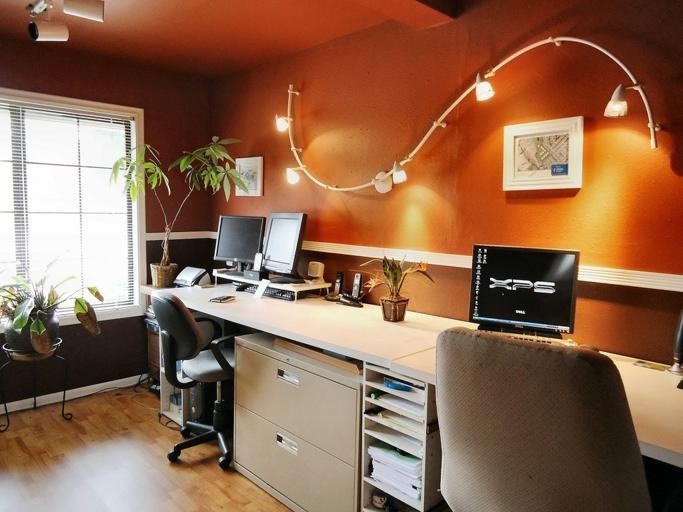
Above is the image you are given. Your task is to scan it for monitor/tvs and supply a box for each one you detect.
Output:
[467,242,580,339]
[213,214,266,276]
[261,211,308,276]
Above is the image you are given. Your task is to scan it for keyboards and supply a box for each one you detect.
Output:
[243,285,307,301]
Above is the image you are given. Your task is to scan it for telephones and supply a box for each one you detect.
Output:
[339,273,363,307]
[325,272,344,301]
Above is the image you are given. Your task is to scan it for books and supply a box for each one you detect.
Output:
[367,375,426,502]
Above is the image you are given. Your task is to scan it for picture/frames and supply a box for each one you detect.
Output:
[502,116,583,192]
[235,156,263,197]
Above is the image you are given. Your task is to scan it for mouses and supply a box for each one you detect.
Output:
[236,284,246,291]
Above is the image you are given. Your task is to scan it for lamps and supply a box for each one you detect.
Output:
[26,0,104,43]
[273,36,664,195]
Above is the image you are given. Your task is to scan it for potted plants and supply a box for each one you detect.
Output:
[359,254,436,322]
[0,260,103,354]
[108,135,249,288]
